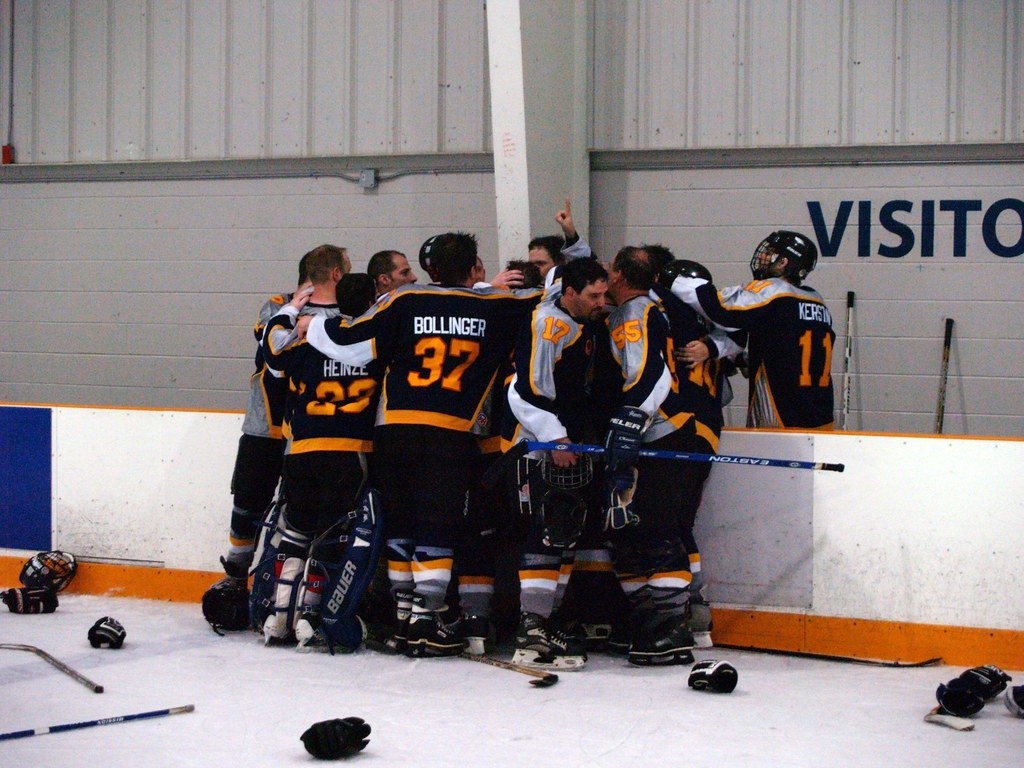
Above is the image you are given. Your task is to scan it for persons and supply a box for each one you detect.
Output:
[220,196,748,662]
[658,230,836,431]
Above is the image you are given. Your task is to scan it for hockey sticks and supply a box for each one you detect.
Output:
[840,291,855,430]
[924,704,976,731]
[457,652,559,688]
[713,643,944,669]
[0,705,195,743]
[935,318,955,435]
[479,439,844,489]
[0,644,104,694]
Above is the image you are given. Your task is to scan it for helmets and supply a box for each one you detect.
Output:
[541,489,587,548]
[19,551,77,593]
[201,580,250,631]
[774,230,817,280]
[675,259,712,285]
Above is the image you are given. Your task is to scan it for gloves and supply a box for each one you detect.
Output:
[936,663,1012,721]
[300,717,371,759]
[88,616,127,649]
[688,658,738,694]
[3,587,59,613]
[605,407,650,466]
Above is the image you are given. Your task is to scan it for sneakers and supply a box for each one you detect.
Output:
[512,627,587,669]
[295,612,329,652]
[629,617,695,665]
[690,595,714,649]
[609,629,635,653]
[386,599,413,652]
[461,616,490,655]
[407,606,464,655]
[263,613,292,646]
